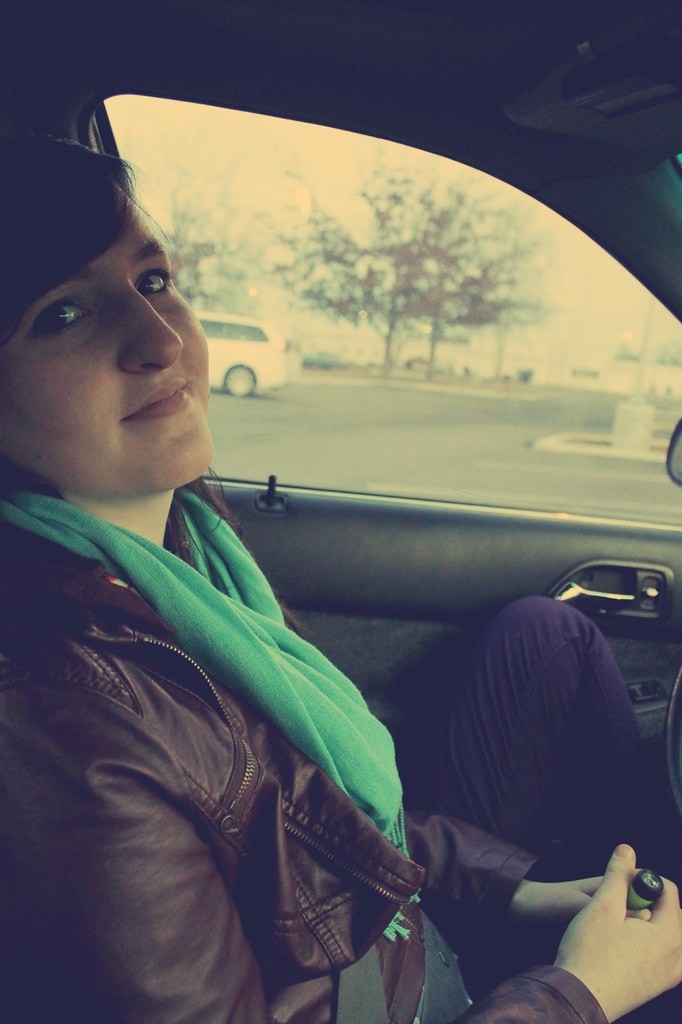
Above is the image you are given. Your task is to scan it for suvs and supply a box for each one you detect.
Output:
[190,309,300,398]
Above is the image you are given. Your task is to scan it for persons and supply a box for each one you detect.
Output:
[0,132,682,1024]
